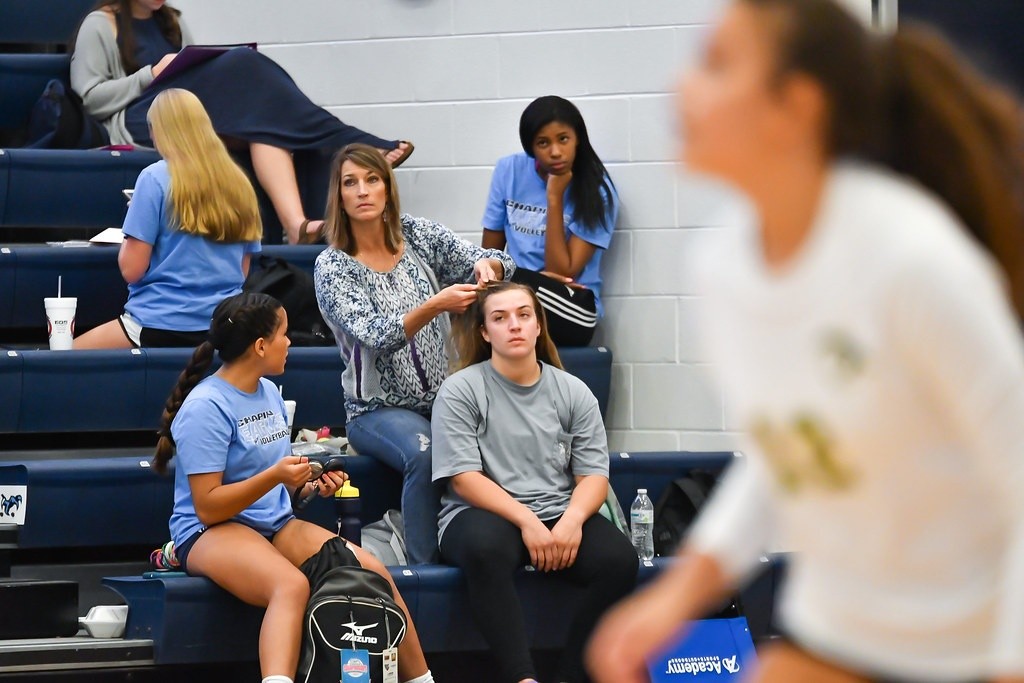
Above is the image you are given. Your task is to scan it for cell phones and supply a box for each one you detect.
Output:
[124,188,134,201]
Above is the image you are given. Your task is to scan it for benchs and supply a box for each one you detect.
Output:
[0,1,789,683]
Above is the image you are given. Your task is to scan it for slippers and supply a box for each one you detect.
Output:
[381,140,414,169]
[296,219,329,246]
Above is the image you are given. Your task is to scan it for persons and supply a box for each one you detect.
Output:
[73,89,262,349]
[482,95,618,347]
[70,1,415,244]
[587,0,1024,683]
[314,143,517,565]
[432,284,638,683]
[152,295,433,683]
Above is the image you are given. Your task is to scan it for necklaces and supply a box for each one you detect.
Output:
[359,253,396,272]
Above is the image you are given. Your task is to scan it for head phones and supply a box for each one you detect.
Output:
[290,456,347,510]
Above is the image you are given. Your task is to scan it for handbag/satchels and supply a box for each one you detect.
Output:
[598,481,632,544]
[360,509,408,566]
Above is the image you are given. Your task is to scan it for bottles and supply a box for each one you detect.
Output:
[631,489,654,561]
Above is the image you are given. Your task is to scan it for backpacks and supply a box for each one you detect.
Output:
[25,79,110,150]
[653,469,743,619]
[242,257,337,347]
[293,522,408,683]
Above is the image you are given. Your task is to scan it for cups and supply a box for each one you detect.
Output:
[44,298,77,351]
[282,400,296,443]
